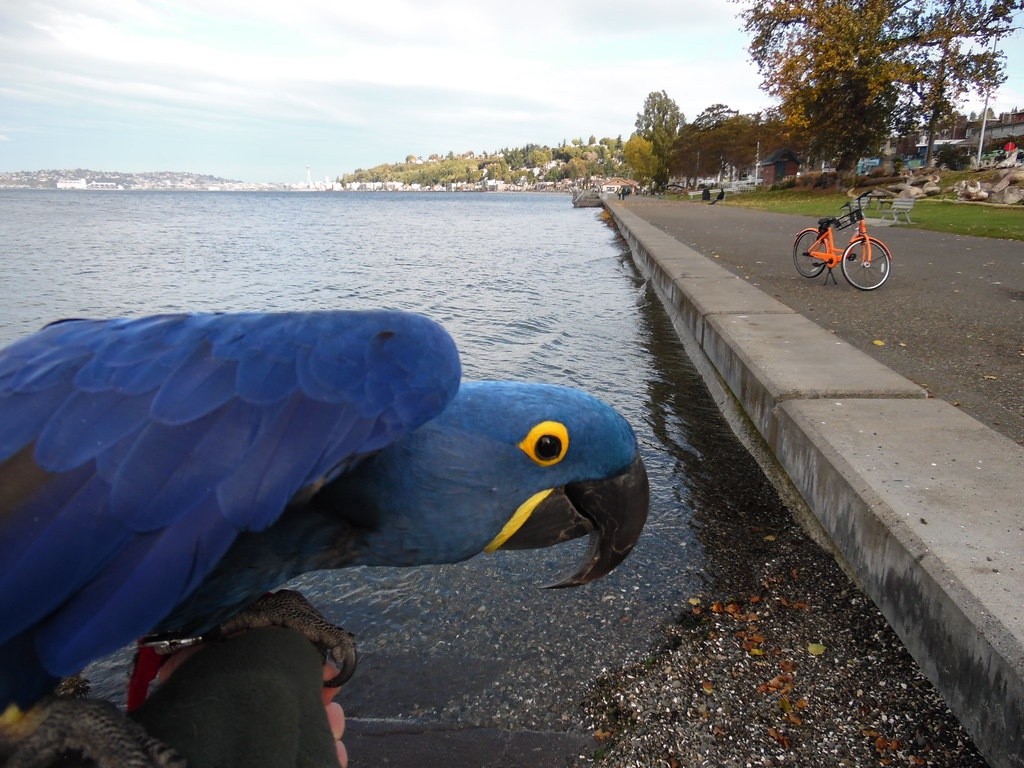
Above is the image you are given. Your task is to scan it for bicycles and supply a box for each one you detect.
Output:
[792,190,893,291]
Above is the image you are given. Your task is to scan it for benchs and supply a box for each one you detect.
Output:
[877,198,916,226]
[841,197,868,222]
[713,195,725,205]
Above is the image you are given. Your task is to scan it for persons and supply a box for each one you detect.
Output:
[79,626,349,768]
[615,187,632,201]
[708,188,724,205]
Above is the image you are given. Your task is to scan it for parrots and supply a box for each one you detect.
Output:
[1,306,650,768]
